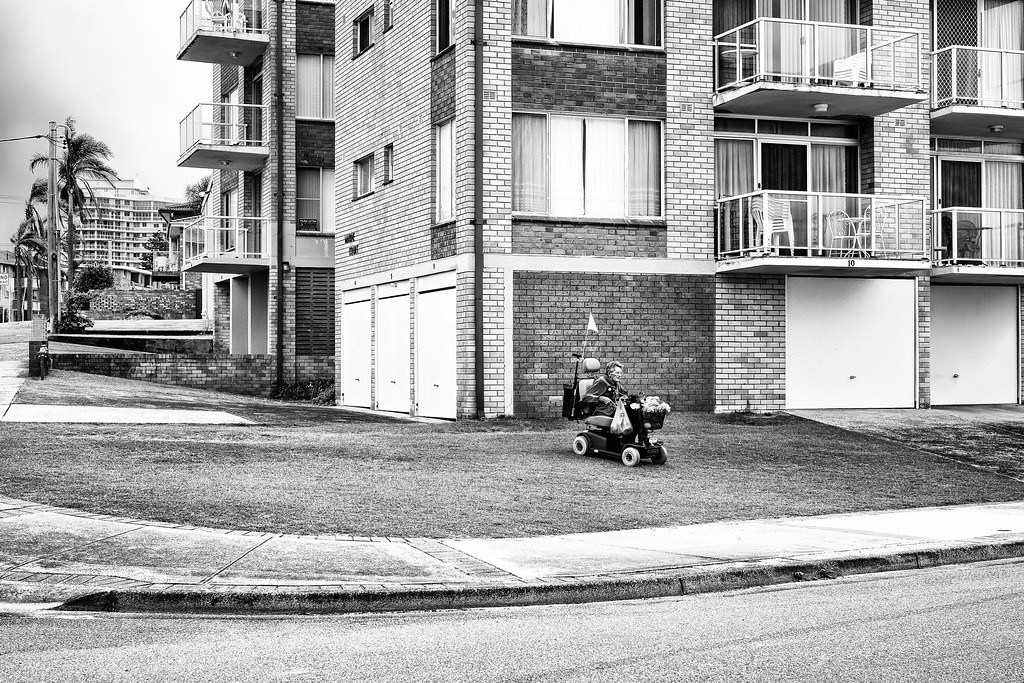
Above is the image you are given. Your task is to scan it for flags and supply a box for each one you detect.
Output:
[588,311,598,333]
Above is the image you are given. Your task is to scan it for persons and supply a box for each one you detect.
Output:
[581,361,649,447]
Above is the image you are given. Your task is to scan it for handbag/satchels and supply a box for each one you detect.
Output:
[610,398,633,435]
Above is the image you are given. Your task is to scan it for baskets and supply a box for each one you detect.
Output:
[643,410,667,429]
